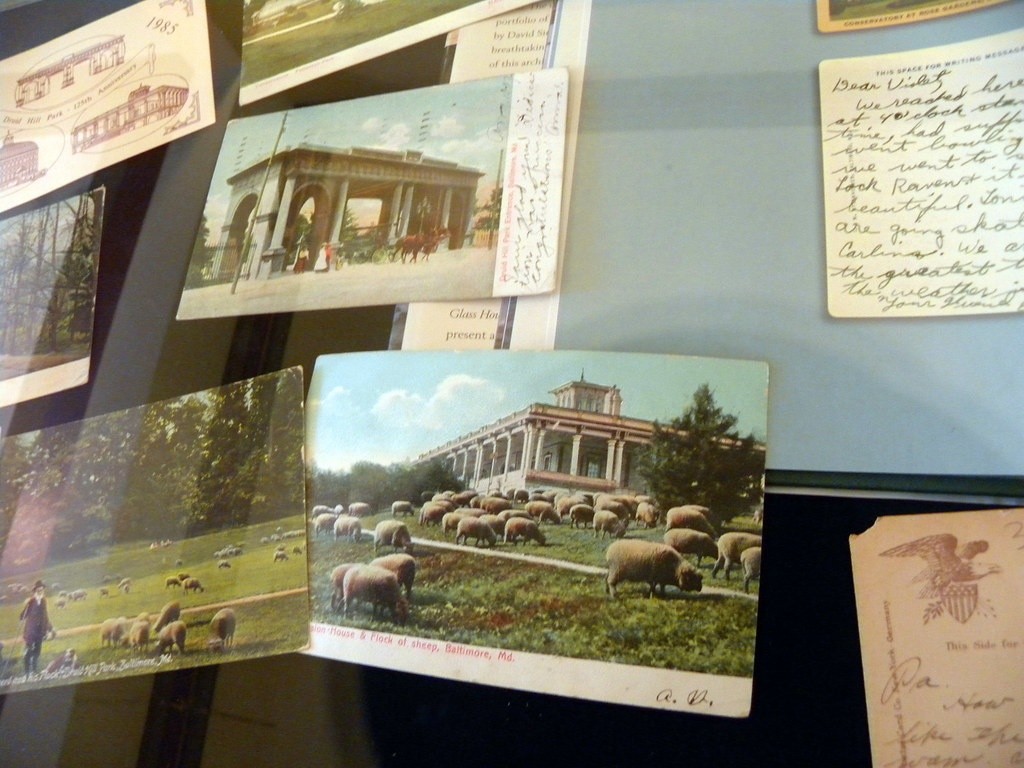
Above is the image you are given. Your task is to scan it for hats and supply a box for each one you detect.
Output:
[32,580,44,591]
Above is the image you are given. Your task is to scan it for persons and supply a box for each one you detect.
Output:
[299,242,332,273]
[19,580,53,675]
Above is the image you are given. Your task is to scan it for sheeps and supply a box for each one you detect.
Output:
[1,522,307,678]
[311,488,762,629]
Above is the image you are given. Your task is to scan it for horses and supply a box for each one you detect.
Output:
[391,225,451,264]
